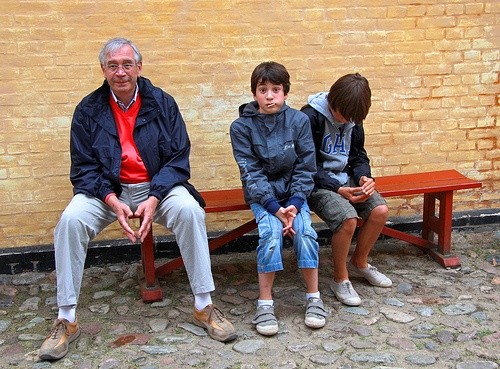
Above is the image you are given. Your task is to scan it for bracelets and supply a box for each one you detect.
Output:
[105,193,116,203]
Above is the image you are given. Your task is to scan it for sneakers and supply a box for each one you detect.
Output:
[330,277,362,305]
[38,313,81,359]
[348,258,392,288]
[192,303,238,342]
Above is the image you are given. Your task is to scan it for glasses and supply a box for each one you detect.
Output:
[104,64,136,73]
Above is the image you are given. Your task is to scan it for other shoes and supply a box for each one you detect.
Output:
[252,299,279,335]
[305,297,329,329]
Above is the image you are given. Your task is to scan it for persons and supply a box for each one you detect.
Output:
[39,38,237,361]
[299,73,392,306]
[230,61,327,336]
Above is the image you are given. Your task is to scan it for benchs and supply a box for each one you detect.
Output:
[125,169,481,302]
